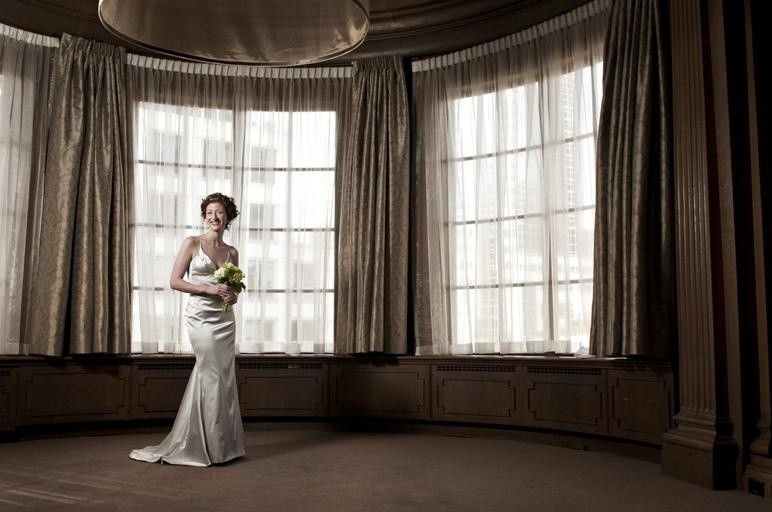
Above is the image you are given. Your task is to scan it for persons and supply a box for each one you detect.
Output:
[128,193,246,467]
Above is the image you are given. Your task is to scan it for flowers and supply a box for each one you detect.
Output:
[212,262,246,313]
